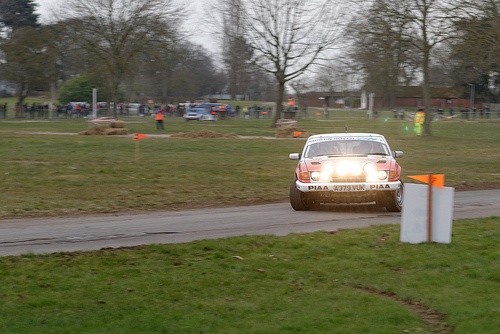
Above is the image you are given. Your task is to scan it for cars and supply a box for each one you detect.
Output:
[289,133,407,213]
[61,100,236,121]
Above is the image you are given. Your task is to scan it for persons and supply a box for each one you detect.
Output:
[2,96,299,119]
[357,141,373,153]
[154,105,165,130]
[414,107,425,137]
[391,105,492,121]
